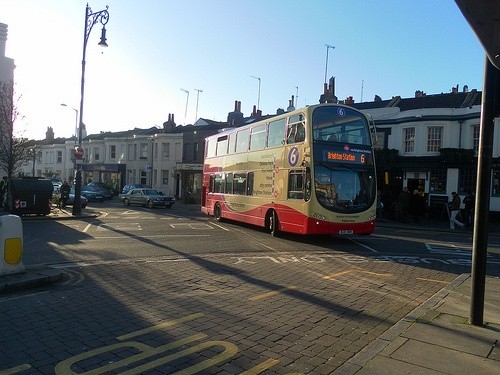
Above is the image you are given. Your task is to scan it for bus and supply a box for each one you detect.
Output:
[200,103,379,236]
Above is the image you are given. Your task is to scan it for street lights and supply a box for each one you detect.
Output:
[293,86,299,108]
[360,80,365,103]
[179,87,189,125]
[195,88,204,122]
[147,135,156,190]
[72,2,110,213]
[323,42,336,89]
[61,102,78,149]
[250,75,261,110]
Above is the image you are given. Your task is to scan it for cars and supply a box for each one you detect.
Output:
[81,186,105,202]
[52,179,116,204]
[122,184,144,195]
[119,186,175,209]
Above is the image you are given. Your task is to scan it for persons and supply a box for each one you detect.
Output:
[399,187,409,222]
[0,176,8,208]
[60,180,71,209]
[410,190,420,226]
[446,192,464,232]
[463,190,475,228]
